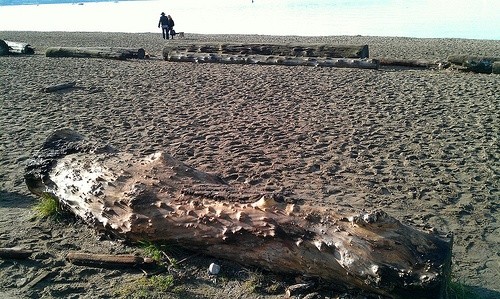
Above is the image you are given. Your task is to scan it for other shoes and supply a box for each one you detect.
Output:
[170,38,173,40]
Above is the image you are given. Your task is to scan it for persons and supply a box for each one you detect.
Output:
[167,14,174,39]
[158,12,170,40]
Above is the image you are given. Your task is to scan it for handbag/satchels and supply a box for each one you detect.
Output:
[169,29,175,35]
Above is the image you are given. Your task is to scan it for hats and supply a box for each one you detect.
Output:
[161,12,165,15]
